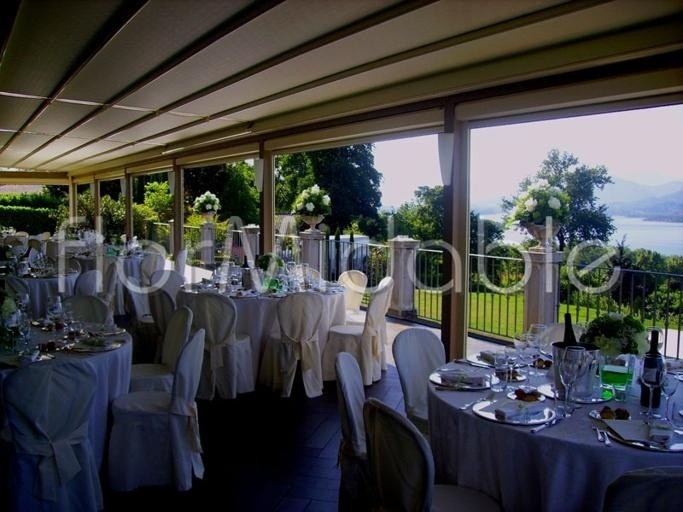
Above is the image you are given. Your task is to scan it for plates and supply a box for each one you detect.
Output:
[465,353,526,370]
[67,337,126,353]
[470,400,557,424]
[228,293,257,298]
[89,326,125,335]
[606,428,682,452]
[180,283,216,289]
[429,372,499,392]
[537,380,615,402]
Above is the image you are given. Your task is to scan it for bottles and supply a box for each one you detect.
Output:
[240,255,249,286]
[561,313,579,348]
[639,329,663,405]
[18,244,32,262]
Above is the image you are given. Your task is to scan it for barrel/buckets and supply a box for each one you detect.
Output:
[550,341,602,392]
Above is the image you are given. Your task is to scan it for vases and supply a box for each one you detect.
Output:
[198,211,216,224]
[296,213,325,232]
[521,223,565,251]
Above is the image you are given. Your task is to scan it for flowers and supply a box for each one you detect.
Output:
[291,186,332,214]
[516,182,570,224]
[192,191,222,213]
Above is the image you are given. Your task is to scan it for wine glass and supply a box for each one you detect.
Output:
[5,293,77,354]
[553,344,588,416]
[7,251,48,276]
[489,320,550,396]
[212,262,318,292]
[639,349,683,429]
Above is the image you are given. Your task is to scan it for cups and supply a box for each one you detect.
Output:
[613,381,625,401]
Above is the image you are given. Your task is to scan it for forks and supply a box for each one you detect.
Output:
[591,422,611,447]
